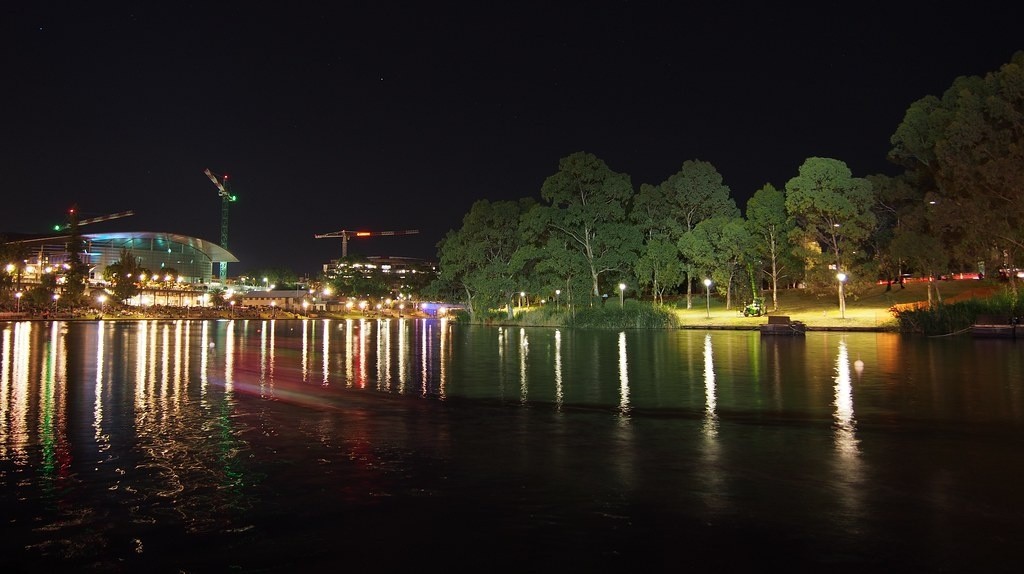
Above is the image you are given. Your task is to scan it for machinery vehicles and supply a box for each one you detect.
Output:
[744,263,767,317]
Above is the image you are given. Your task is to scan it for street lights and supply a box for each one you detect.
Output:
[838,273,846,319]
[620,284,625,311]
[15,293,21,313]
[303,303,308,317]
[703,279,711,319]
[53,294,60,313]
[99,295,106,311]
[230,301,236,316]
[271,301,276,315]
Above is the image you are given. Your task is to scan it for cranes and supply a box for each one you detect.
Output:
[204,168,236,290]
[314,227,422,259]
[51,208,135,236]
